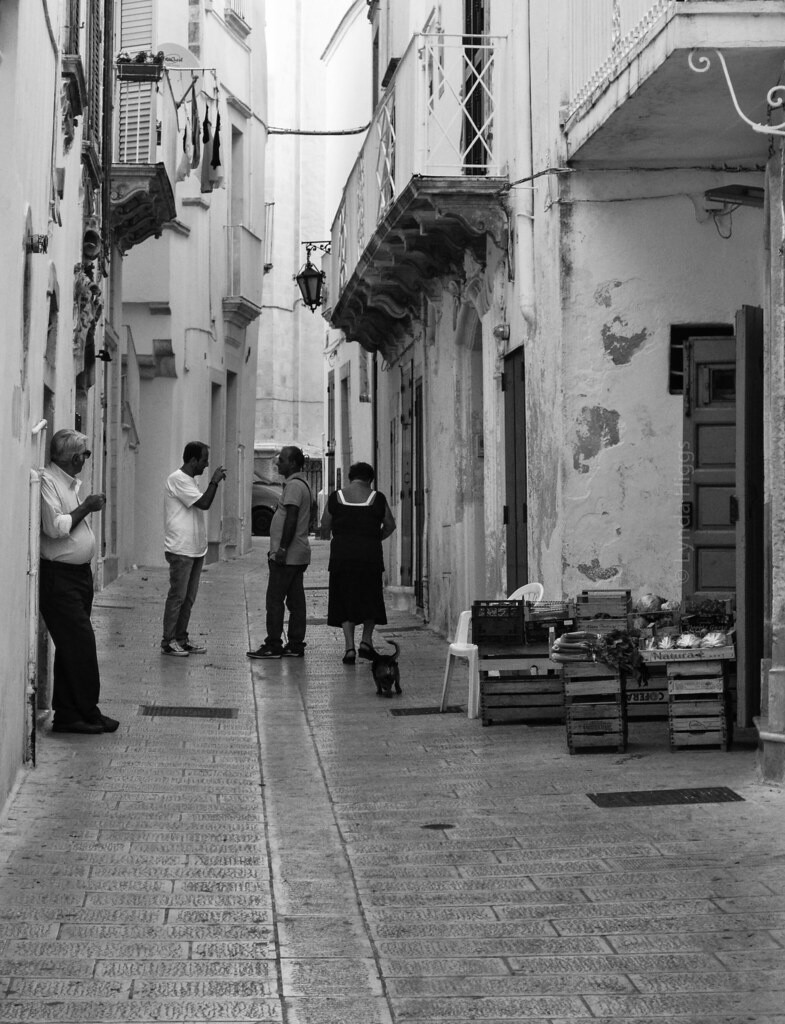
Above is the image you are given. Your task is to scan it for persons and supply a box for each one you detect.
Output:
[244,443,314,661]
[314,463,397,666]
[158,437,226,657]
[40,427,121,737]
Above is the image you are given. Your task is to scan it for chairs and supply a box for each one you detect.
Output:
[440,583,545,718]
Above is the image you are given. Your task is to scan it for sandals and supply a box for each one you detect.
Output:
[358,641,377,660]
[342,648,356,664]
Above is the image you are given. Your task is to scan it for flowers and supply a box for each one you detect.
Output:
[118,49,165,63]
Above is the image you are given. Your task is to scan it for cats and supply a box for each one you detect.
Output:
[358,640,404,696]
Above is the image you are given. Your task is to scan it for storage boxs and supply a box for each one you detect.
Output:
[470,589,739,755]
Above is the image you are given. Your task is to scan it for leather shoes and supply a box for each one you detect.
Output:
[51,710,119,734]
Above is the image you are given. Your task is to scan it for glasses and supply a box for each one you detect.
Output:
[79,450,91,459]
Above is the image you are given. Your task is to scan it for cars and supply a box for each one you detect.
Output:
[252,470,317,536]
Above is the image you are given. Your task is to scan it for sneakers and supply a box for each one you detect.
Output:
[247,644,305,659]
[160,638,208,657]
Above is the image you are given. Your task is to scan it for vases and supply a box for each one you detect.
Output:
[120,65,160,81]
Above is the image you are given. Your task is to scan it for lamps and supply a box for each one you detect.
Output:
[95,349,112,362]
[294,241,329,310]
